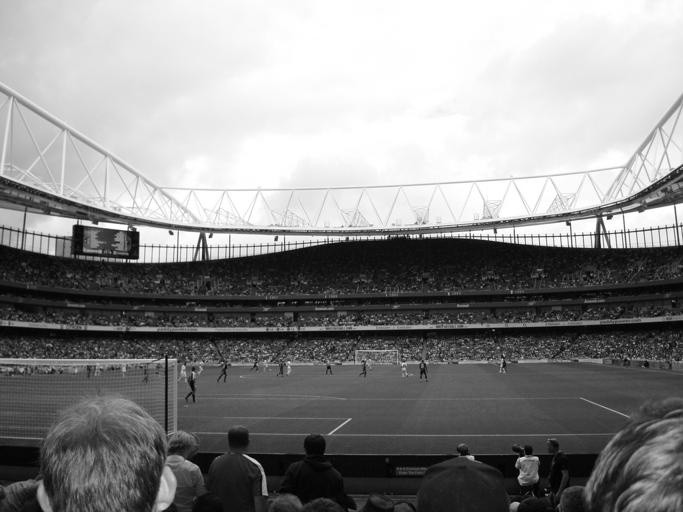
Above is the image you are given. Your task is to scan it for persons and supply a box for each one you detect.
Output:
[160,424,268,512]
[70,345,432,405]
[363,434,586,512]
[498,351,508,377]
[268,432,356,510]
[35,393,176,512]
[1,478,42,512]
[0,253,682,363]
[583,394,683,512]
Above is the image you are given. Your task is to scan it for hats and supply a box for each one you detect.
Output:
[416,457,508,512]
